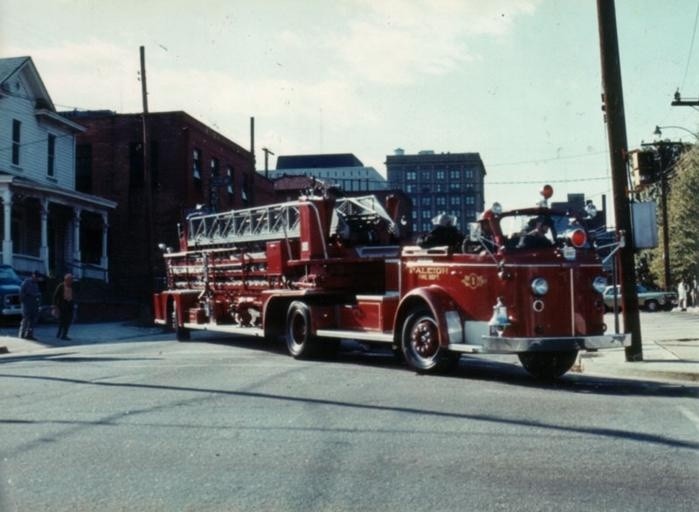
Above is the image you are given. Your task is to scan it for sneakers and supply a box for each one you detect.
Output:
[19,334,37,341]
[56,334,70,340]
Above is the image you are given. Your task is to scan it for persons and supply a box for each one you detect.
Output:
[415,211,465,251]
[517,215,554,254]
[676,277,692,311]
[18,269,42,341]
[52,272,78,340]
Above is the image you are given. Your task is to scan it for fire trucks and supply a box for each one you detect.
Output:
[152,175,634,379]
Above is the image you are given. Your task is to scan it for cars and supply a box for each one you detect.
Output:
[0,263,43,326]
[602,283,678,313]
